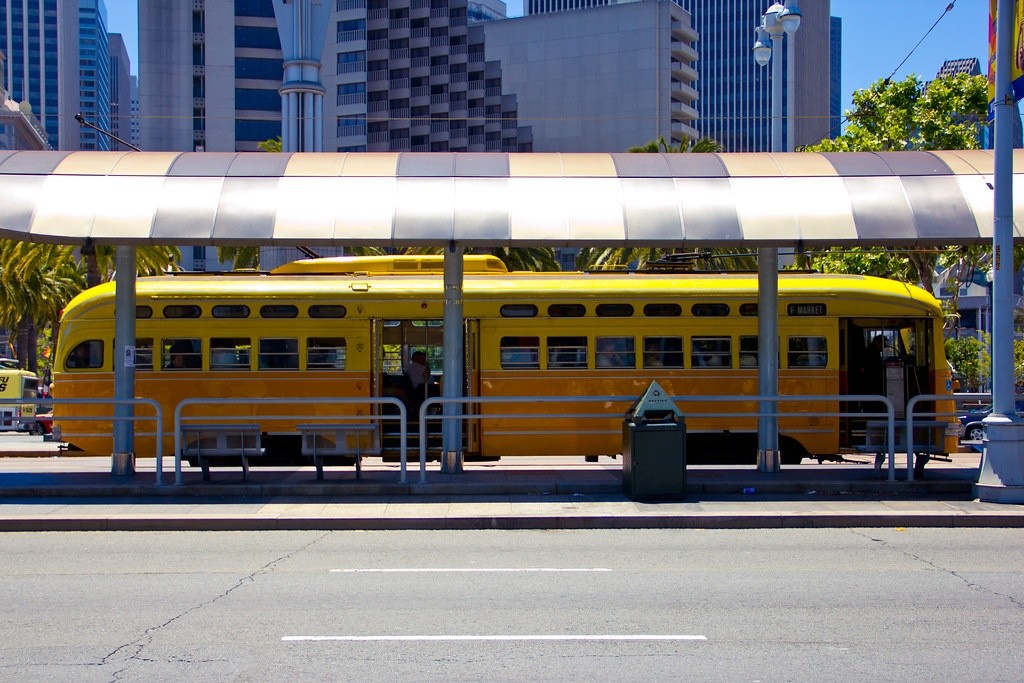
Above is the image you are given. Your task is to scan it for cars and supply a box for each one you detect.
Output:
[962,398,1024,440]
[35,408,53,435]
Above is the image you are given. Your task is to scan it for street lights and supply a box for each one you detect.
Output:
[754,4,802,153]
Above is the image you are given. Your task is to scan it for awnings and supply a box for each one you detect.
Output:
[986,0,1024,123]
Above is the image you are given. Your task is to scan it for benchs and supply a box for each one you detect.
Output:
[296,424,380,478]
[180,423,267,479]
[854,420,949,482]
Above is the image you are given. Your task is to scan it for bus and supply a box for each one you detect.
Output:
[0,357,39,433]
[46,254,961,470]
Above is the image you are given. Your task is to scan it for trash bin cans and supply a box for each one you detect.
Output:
[621,378,687,504]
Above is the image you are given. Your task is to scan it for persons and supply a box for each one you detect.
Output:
[863,335,888,408]
[501,338,756,368]
[73,338,337,369]
[410,350,441,419]
